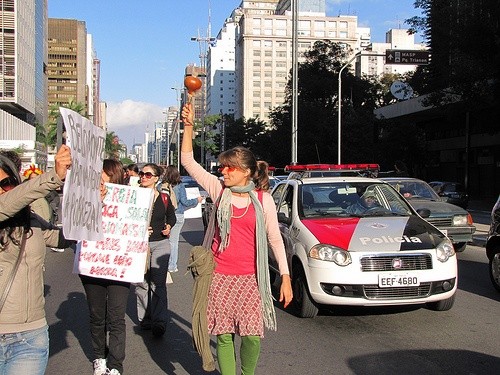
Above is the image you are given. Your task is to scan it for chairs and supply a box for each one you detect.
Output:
[327,190,347,209]
[302,193,320,213]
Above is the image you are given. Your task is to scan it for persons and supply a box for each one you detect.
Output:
[349,190,381,214]
[402,187,412,198]
[181,102,293,375]
[0,145,108,375]
[73,158,205,375]
[23,163,43,181]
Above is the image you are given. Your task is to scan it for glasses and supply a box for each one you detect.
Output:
[138,172,157,178]
[218,167,236,172]
[0,176,19,192]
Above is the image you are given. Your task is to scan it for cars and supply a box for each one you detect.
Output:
[269,163,459,319]
[377,177,476,253]
[485,195,500,292]
[178,167,379,238]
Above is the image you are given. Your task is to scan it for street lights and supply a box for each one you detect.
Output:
[190,37,217,168]
[163,111,169,167]
[171,87,180,173]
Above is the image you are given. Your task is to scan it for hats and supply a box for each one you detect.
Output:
[364,191,375,199]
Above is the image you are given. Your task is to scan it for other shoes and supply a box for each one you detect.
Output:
[152,324,165,341]
[168,266,178,272]
[139,323,151,330]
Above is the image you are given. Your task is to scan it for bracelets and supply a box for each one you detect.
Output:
[183,123,192,126]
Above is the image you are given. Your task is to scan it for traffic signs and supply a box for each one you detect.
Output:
[384,48,432,66]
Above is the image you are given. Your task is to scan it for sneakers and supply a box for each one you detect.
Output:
[105,368,121,375]
[93,359,107,375]
